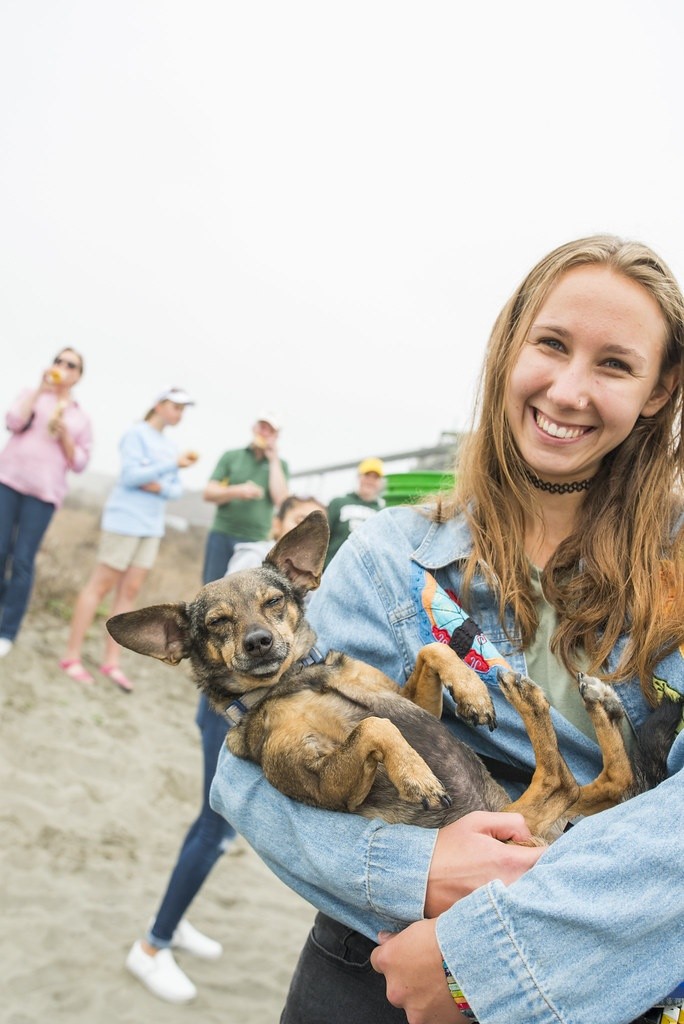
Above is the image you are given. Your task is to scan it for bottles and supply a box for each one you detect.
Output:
[44,369,69,385]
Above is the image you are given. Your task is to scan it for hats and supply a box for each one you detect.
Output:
[357,457,382,477]
[155,386,197,407]
[255,414,280,431]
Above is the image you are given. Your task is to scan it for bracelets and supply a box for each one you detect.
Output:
[440,955,480,1024]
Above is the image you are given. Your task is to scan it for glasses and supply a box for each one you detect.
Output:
[54,357,80,369]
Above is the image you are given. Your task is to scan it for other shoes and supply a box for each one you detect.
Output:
[0,637,12,658]
[127,941,198,1005]
[100,664,132,691]
[149,916,223,962]
[58,657,94,684]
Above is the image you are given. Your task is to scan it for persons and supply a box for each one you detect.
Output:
[321,458,385,573]
[59,378,199,697]
[203,413,291,585]
[0,344,96,657]
[208,235,684,1024]
[126,485,331,1001]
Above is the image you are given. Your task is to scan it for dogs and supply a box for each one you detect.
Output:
[105,508,636,847]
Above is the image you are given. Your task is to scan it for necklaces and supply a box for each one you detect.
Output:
[519,461,594,492]
